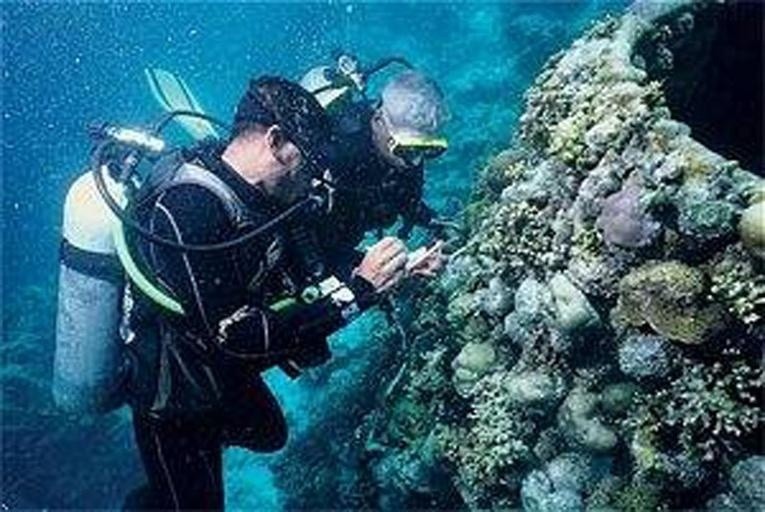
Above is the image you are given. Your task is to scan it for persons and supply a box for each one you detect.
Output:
[122,72,449,512]
[272,72,447,369]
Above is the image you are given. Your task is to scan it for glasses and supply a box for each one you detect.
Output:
[379,107,449,165]
